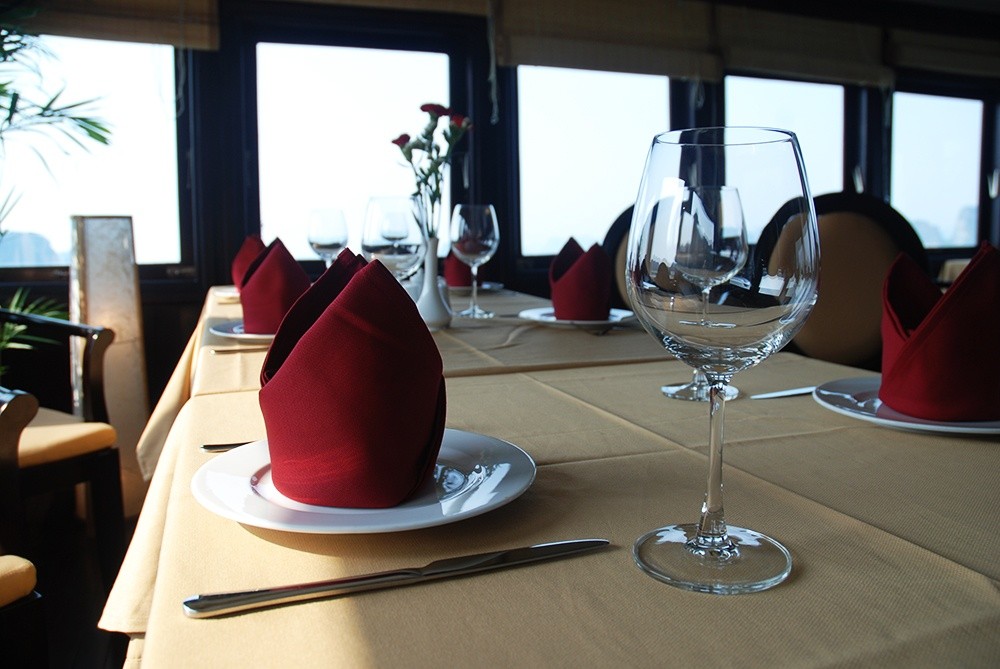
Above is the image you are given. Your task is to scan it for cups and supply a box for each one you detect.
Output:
[360,193,429,284]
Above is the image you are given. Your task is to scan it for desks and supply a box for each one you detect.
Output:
[97,286,1000,669]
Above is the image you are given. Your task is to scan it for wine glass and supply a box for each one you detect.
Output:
[624,126,822,599]
[307,207,348,270]
[666,183,747,330]
[379,209,410,248]
[660,367,740,403]
[449,204,500,319]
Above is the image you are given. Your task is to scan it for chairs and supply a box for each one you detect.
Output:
[604,202,710,312]
[751,193,932,372]
[1,308,126,669]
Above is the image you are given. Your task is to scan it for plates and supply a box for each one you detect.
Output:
[518,306,637,330]
[449,281,503,296]
[208,319,275,345]
[213,286,240,298]
[188,425,538,536]
[812,374,1000,436]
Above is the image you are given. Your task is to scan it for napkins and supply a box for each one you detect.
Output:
[879,240,1000,423]
[259,247,447,510]
[241,238,311,335]
[548,241,612,321]
[232,237,266,291]
[446,249,482,287]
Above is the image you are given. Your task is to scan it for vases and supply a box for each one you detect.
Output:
[416,239,452,332]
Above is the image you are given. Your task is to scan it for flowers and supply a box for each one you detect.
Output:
[391,104,470,239]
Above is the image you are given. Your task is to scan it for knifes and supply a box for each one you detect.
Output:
[184,537,611,621]
[750,386,816,399]
